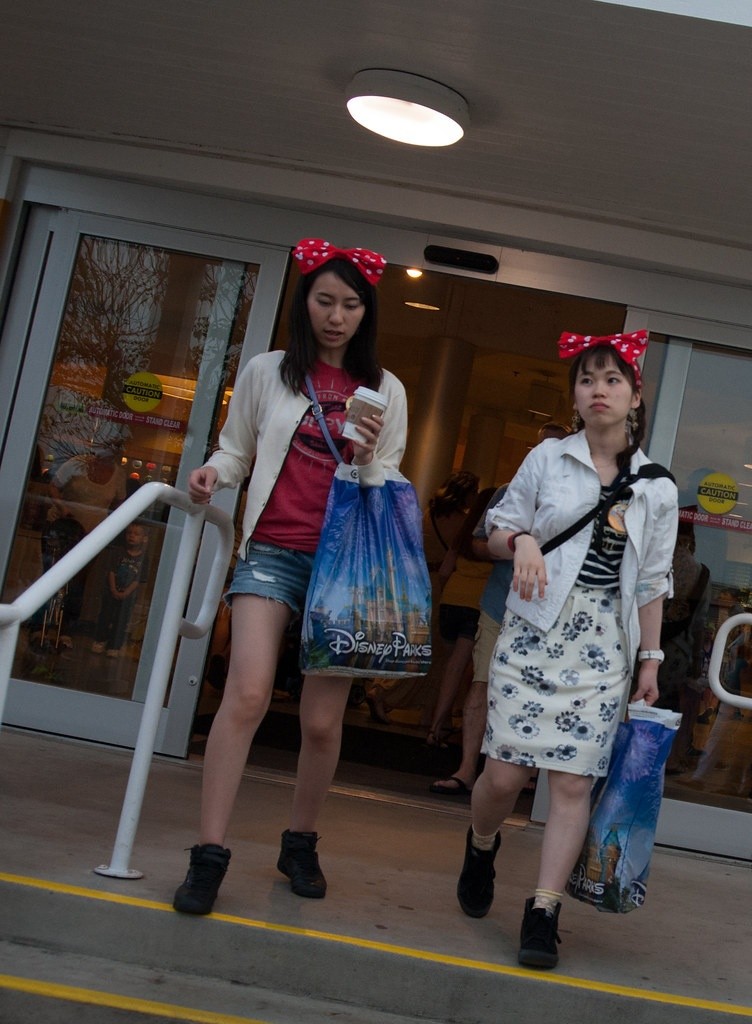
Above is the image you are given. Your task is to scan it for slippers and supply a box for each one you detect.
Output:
[428,775,472,796]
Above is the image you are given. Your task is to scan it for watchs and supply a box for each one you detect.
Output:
[638,649,664,664]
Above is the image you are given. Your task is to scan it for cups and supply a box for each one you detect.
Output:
[341,386,391,444]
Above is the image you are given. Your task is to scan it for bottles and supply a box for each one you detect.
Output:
[599,823,622,888]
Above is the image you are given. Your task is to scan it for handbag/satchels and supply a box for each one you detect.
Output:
[563,698,683,914]
[299,459,433,679]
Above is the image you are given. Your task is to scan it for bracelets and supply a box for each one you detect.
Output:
[508,531,531,552]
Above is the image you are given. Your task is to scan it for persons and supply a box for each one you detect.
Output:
[659,468,752,799]
[421,329,678,968]
[172,240,406,915]
[30,428,150,659]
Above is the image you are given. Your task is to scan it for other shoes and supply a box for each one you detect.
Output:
[106,646,121,659]
[29,630,47,650]
[422,729,443,751]
[91,638,107,654]
[50,636,73,650]
[365,686,384,721]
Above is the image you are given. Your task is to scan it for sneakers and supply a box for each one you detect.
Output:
[456,825,502,918]
[173,843,231,916]
[517,896,562,971]
[277,829,327,899]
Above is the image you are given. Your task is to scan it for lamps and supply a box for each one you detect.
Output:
[405,270,441,310]
[345,69,470,147]
[526,379,565,417]
